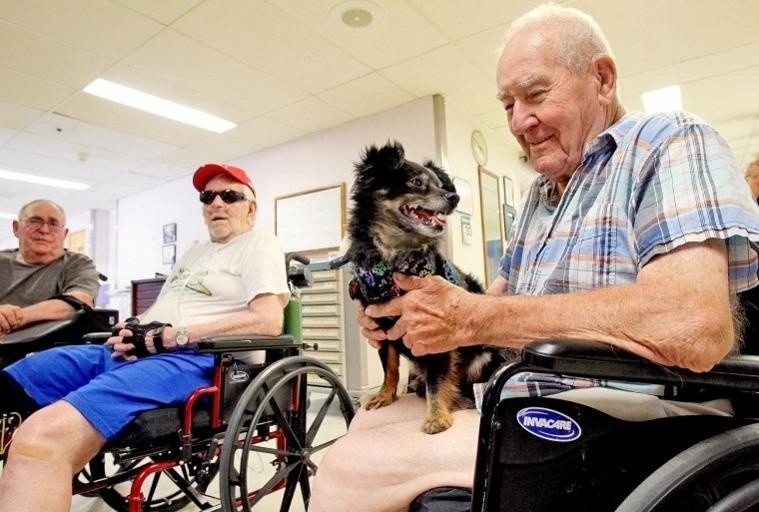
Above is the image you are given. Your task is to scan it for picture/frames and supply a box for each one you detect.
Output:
[273,182,346,257]
[452,175,473,245]
[503,174,515,206]
[161,222,176,264]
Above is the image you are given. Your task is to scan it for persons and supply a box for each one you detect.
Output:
[0,199,103,332]
[303,1,759,512]
[2,162,294,512]
[744,158,759,201]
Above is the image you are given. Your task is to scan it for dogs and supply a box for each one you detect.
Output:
[347,138,520,435]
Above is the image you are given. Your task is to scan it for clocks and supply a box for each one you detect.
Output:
[471,129,489,167]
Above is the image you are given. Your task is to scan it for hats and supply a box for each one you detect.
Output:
[193,164,256,200]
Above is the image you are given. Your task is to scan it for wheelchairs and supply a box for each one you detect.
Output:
[412,332,758,511]
[90,250,357,511]
[0,306,144,499]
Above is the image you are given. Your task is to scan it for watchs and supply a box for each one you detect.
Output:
[175,324,190,350]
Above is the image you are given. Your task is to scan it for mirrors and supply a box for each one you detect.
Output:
[478,165,504,289]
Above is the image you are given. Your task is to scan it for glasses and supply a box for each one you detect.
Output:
[19,218,64,234]
[200,189,252,206]
